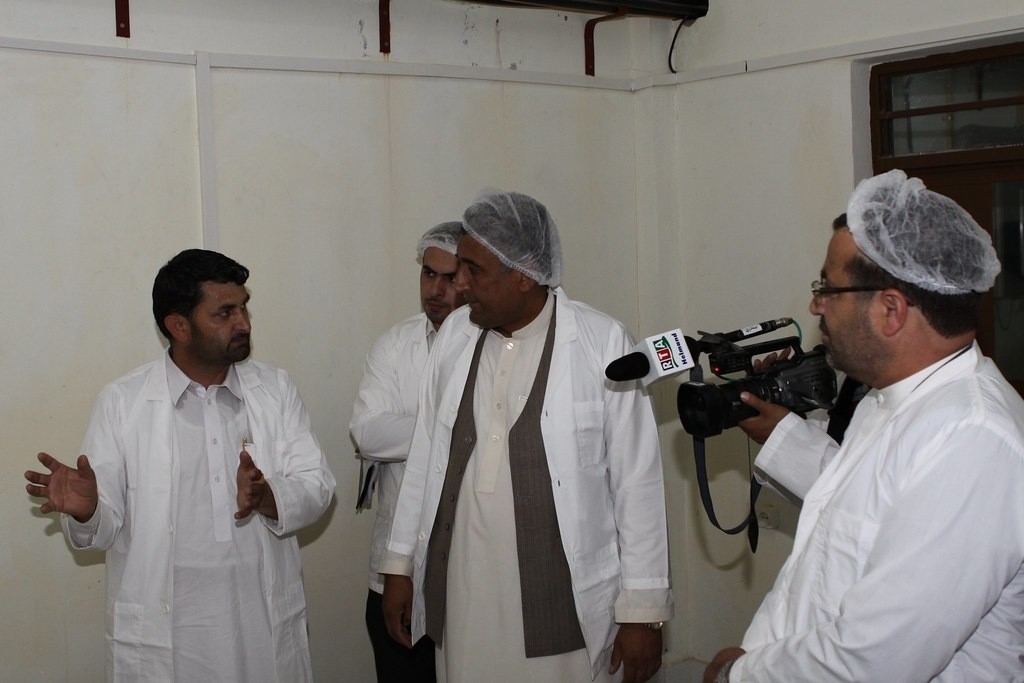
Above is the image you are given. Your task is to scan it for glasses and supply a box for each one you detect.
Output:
[811,281,915,307]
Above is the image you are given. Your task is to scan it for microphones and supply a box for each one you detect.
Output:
[605,317,793,387]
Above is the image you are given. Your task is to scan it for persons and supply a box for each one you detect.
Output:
[377,190,673,683]
[701,169,1024,683]
[24,248,338,683]
[349,219,471,681]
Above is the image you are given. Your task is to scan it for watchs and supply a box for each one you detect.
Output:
[637,620,663,631]
[713,660,733,683]
